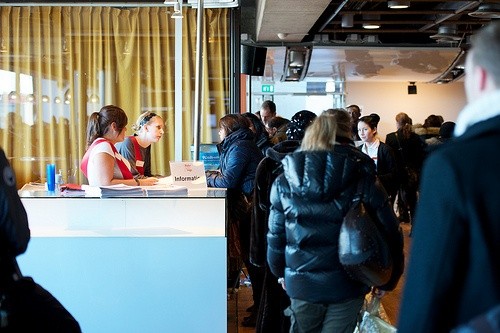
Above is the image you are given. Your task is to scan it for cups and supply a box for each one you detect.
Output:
[46,164,56,191]
[68,169,77,184]
[56,180,65,189]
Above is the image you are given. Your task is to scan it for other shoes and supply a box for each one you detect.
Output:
[241,312,256,327]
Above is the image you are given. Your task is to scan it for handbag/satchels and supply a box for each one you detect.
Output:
[10,275,81,332]
[352,295,397,333]
[339,199,393,285]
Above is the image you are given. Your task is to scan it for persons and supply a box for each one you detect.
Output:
[134,179,140,185]
[81,106,158,186]
[397,24,500,333]
[113,110,166,178]
[0,146,32,333]
[206,100,456,333]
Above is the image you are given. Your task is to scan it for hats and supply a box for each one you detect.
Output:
[285,110,316,139]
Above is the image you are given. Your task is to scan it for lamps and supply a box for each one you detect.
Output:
[429,24,466,44]
[363,24,382,30]
[388,0,410,8]
[342,15,353,27]
[468,4,500,19]
[171,3,184,19]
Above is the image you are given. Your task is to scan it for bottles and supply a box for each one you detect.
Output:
[55,174,62,183]
